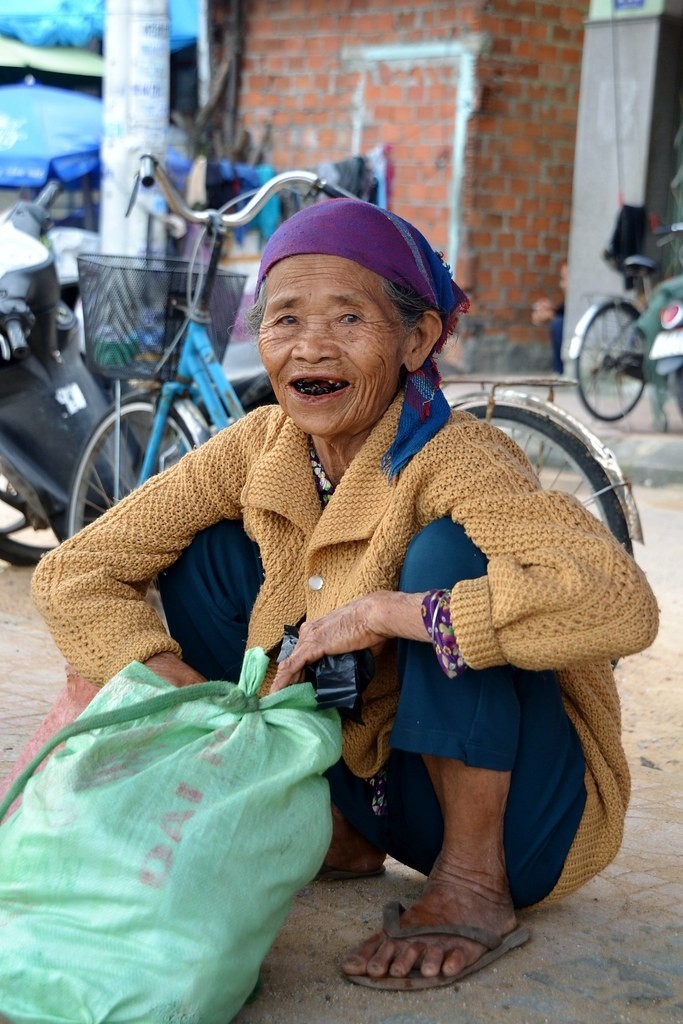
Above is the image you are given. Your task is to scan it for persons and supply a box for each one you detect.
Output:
[30,197,660,991]
[528,258,567,380]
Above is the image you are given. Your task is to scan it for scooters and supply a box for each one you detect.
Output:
[648,301,683,418]
[0,181,157,567]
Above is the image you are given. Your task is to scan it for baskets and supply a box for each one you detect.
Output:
[78,253,248,381]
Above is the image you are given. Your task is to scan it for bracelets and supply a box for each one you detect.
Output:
[431,588,450,650]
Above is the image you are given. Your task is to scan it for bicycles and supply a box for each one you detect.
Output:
[68,149,646,670]
[568,222,683,422]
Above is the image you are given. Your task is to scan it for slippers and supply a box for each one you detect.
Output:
[342,902,531,990]
[315,865,386,880]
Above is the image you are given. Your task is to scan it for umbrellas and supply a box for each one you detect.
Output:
[0,74,102,188]
